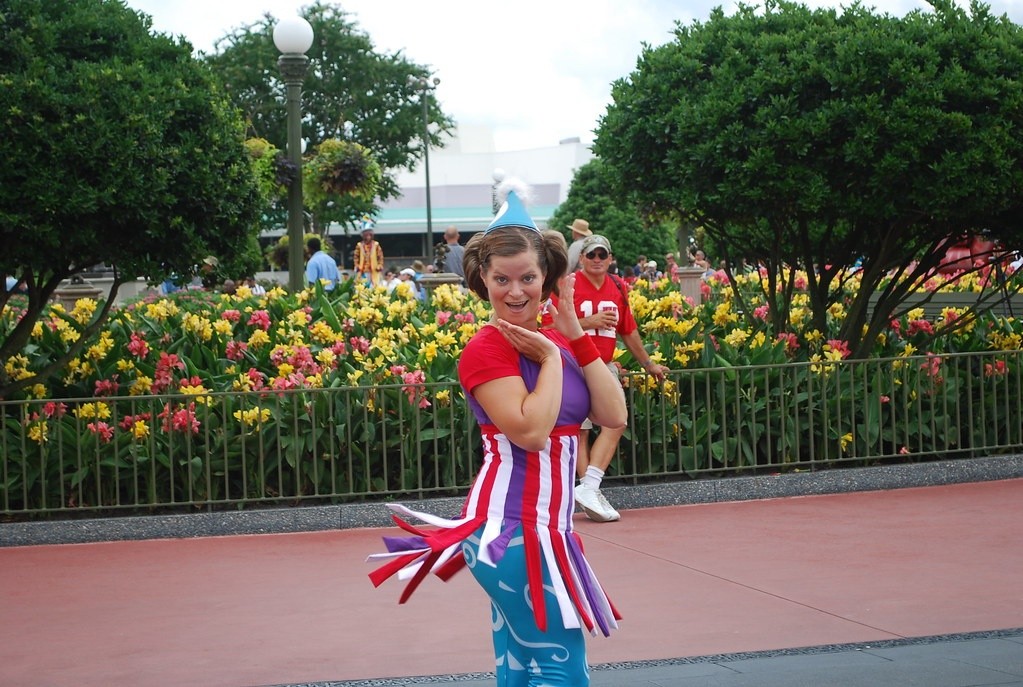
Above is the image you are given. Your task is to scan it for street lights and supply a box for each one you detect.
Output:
[273,15,314,297]
[404,75,440,266]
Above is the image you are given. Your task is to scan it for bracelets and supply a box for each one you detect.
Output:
[643,359,652,368]
[569,332,601,366]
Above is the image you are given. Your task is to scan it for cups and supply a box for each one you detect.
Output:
[603,307,617,331]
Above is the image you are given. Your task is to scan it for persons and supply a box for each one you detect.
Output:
[162,255,265,295]
[307,237,341,293]
[694,250,790,277]
[566,219,592,274]
[365,179,623,687]
[6,269,28,295]
[541,235,670,522]
[609,253,678,283]
[354,220,469,298]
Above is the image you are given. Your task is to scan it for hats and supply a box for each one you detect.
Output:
[648,261,657,268]
[399,268,416,277]
[412,260,425,273]
[566,219,593,237]
[203,256,219,268]
[581,235,611,256]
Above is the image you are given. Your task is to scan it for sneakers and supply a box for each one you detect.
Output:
[595,489,621,521]
[574,485,607,522]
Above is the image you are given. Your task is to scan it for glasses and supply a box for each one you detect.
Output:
[586,252,608,260]
